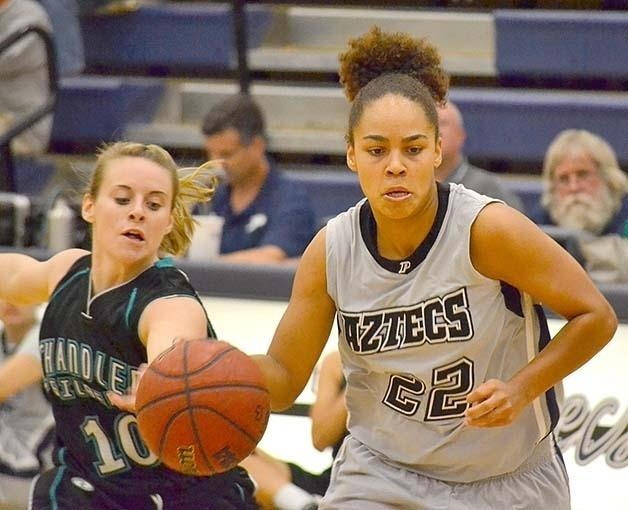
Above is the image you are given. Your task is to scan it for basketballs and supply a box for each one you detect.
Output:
[137,338,271,477]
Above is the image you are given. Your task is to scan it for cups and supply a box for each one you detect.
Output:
[184,214,225,262]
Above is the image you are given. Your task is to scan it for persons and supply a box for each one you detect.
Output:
[187,92,317,264]
[3,1,58,154]
[538,128,627,285]
[433,100,526,212]
[248,28,619,509]
[0,137,352,507]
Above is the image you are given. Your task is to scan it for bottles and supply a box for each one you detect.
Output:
[46,195,73,256]
